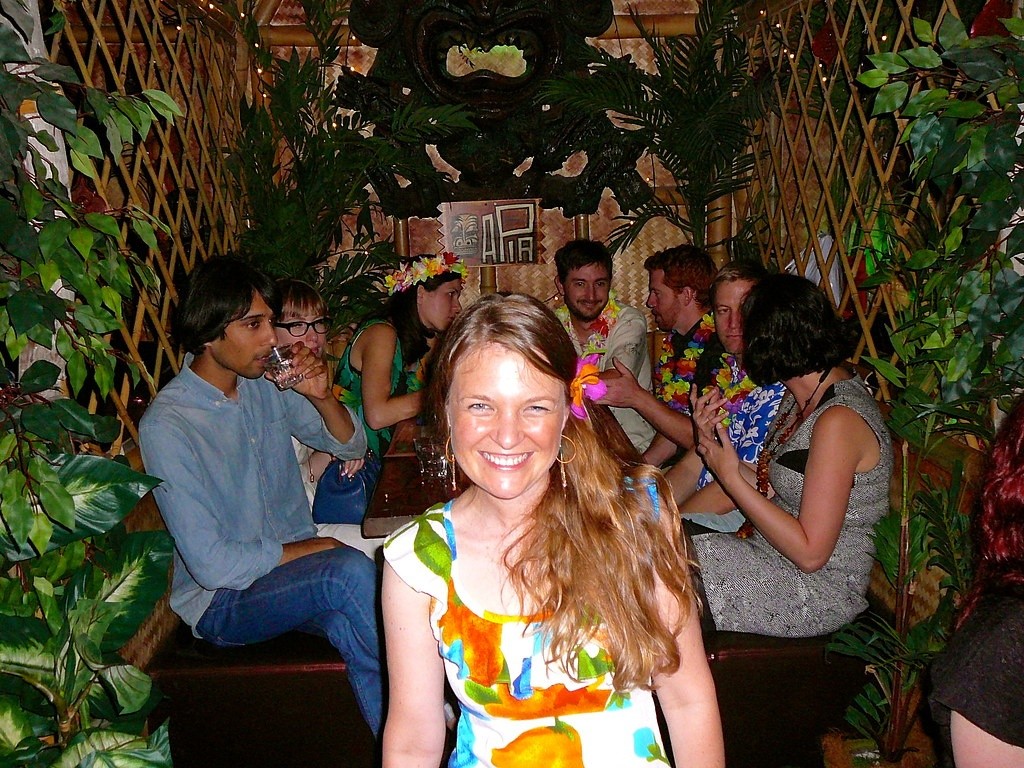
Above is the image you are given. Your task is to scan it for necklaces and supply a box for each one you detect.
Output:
[735,365,834,539]
[656,310,717,417]
[702,352,758,426]
[550,290,620,362]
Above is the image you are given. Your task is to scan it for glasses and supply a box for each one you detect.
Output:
[273,317,333,337]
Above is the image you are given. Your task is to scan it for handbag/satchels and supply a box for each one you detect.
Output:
[311,444,383,525]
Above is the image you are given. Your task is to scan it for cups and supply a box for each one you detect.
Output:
[414,437,448,477]
[260,344,303,391]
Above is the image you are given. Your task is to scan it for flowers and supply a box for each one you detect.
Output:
[570,353,609,420]
[384,250,469,296]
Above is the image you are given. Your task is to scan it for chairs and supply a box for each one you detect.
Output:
[99,436,374,768]
[700,400,992,768]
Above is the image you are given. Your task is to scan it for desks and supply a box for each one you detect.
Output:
[363,401,641,538]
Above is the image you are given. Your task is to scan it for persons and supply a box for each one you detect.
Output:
[380,289,727,768]
[137,241,893,741]
